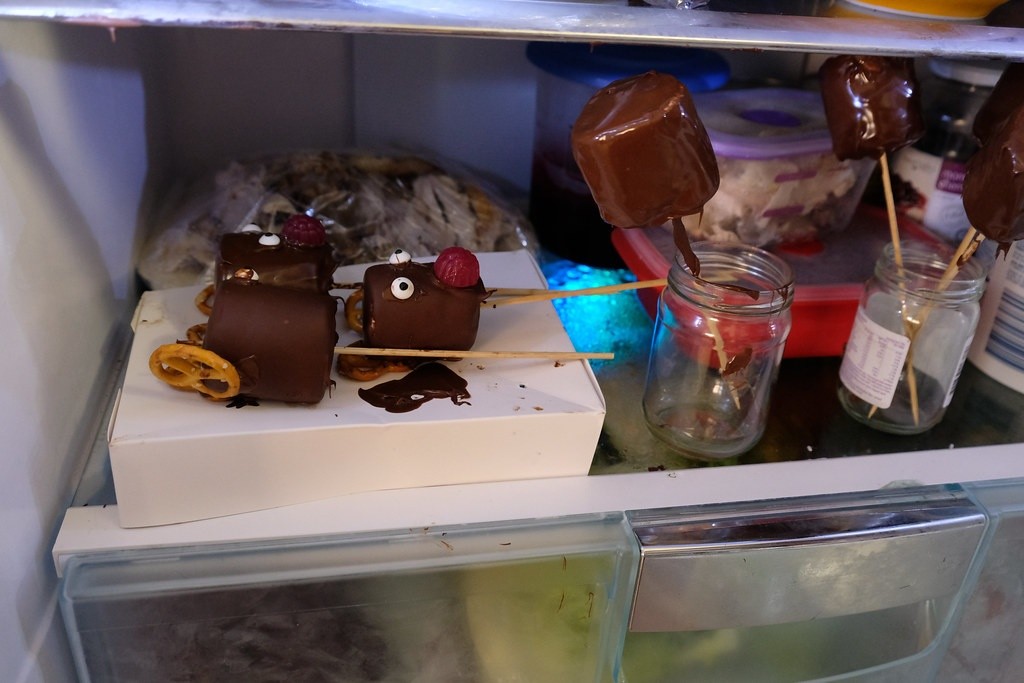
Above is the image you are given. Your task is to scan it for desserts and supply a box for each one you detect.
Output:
[818,52,929,160]
[571,74,721,230]
[144,218,486,404]
[959,60,1024,245]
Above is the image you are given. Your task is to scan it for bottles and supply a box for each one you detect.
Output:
[839,239,986,433]
[642,241,794,459]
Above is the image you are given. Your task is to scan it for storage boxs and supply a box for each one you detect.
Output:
[93,248,608,529]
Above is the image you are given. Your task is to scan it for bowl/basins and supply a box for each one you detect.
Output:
[824,0,1006,20]
[681,87,877,246]
